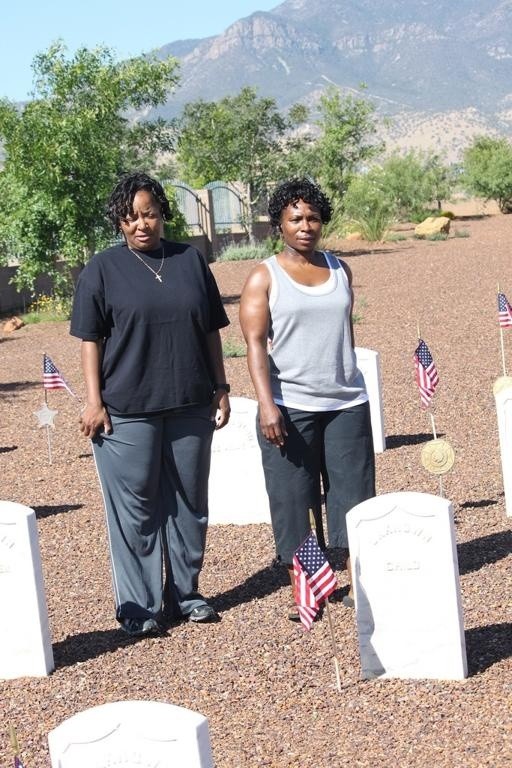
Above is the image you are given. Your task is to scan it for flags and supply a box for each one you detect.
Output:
[293,529,338,632]
[413,338,440,412]
[42,354,76,397]
[498,292,512,330]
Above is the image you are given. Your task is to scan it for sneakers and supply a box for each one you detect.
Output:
[121,617,161,635]
[188,604,215,621]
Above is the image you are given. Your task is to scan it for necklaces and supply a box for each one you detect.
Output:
[128,243,164,284]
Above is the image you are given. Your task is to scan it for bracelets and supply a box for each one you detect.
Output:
[211,383,231,393]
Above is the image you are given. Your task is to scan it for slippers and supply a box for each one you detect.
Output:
[288,601,300,618]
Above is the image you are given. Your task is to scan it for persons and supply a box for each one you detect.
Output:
[69,171,233,639]
[238,176,376,621]
